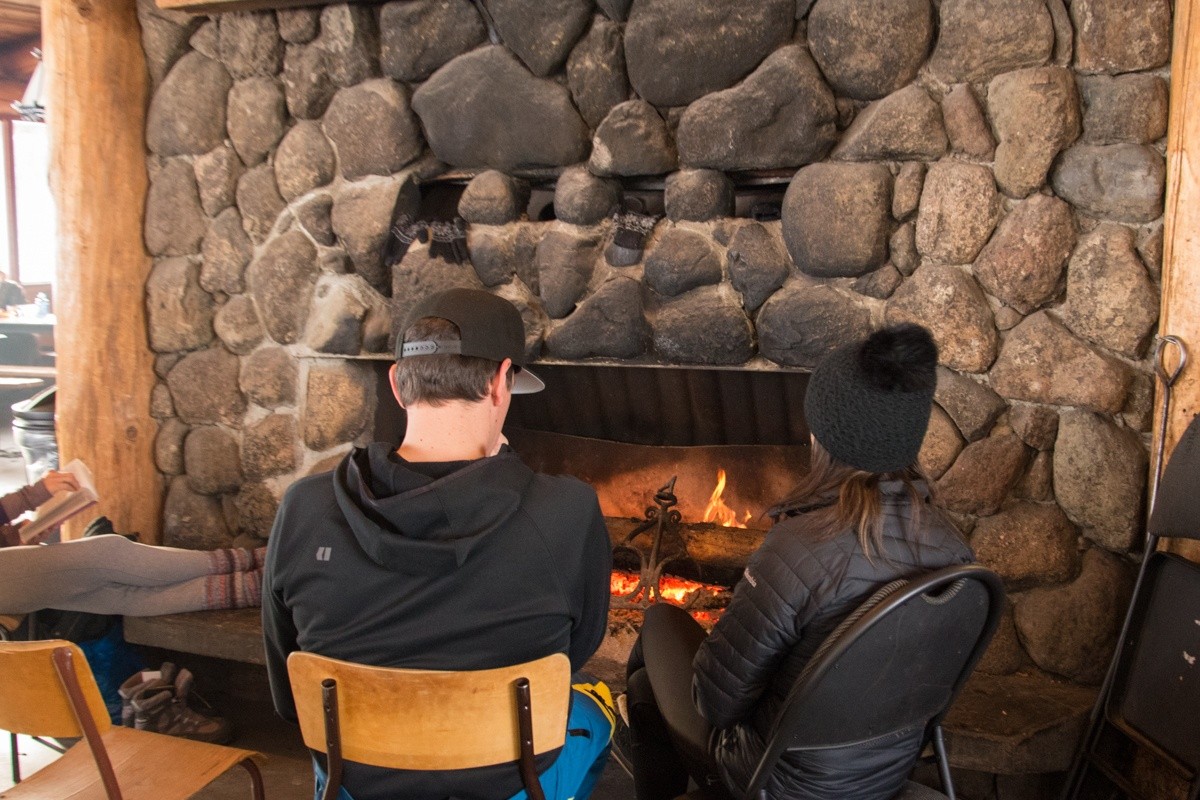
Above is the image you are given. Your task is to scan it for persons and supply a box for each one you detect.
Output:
[1,473,274,628]
[0,271,40,366]
[624,326,983,800]
[258,285,617,800]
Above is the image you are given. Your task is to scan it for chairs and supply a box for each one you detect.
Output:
[676,561,1004,800]
[1056,414,1200,800]
[0,639,270,800]
[287,650,571,800]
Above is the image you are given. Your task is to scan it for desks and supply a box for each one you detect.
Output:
[124,607,264,665]
[0,313,52,334]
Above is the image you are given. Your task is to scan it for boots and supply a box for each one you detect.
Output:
[117,662,230,745]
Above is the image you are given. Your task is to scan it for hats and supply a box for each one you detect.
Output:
[396,288,545,394]
[803,322,938,472]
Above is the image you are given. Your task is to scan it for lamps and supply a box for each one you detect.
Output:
[9,46,46,123]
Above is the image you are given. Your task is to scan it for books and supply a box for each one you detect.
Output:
[14,458,97,546]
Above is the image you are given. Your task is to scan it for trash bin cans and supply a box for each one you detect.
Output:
[9,384,59,486]
